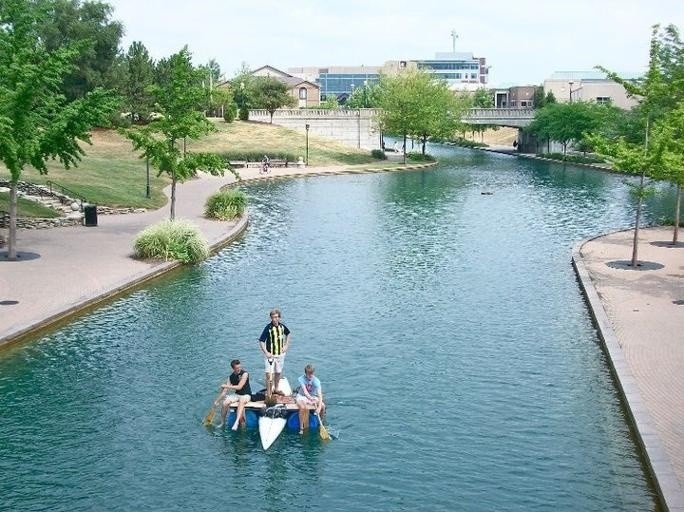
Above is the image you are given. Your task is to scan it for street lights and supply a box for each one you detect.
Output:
[568,81,583,104]
[305,124,310,165]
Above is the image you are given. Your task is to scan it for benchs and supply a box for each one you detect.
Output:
[267,156,287,169]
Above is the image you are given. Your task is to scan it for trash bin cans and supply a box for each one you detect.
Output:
[85,205,97,226]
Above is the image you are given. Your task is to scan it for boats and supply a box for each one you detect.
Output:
[226,387,325,433]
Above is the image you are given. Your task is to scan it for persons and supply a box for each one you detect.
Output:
[296,364,326,434]
[258,310,290,399]
[215,360,252,431]
[262,155,269,163]
[394,141,399,153]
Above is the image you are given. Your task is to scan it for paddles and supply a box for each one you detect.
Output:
[313,400,328,439]
[204,388,225,425]
